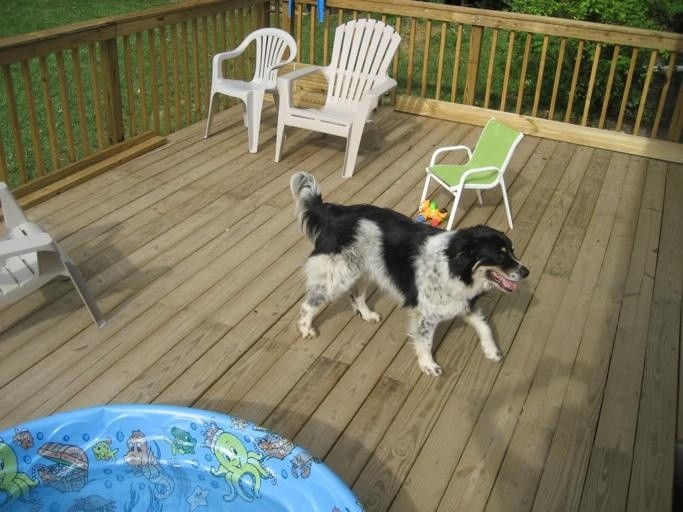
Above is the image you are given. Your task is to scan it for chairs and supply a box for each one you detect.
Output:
[204,28,297,154]
[419,118,523,230]
[275,18,401,179]
[0,181,106,329]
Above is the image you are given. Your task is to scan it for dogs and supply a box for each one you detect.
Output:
[289,170,532,379]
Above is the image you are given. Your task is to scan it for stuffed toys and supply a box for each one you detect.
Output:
[416,198,448,228]
